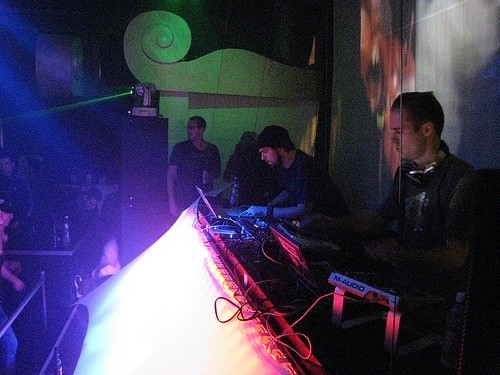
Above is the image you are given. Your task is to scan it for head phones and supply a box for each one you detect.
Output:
[397,143,449,188]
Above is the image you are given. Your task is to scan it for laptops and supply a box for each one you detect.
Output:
[194,187,247,217]
[269,226,334,296]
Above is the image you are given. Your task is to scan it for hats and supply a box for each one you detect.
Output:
[257,125,295,148]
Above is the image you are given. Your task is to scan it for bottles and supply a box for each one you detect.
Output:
[61,215,71,249]
[230,181,240,209]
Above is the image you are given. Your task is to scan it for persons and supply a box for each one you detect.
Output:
[166,116,221,217]
[0,141,123,375]
[304,91,479,375]
[238,125,349,230]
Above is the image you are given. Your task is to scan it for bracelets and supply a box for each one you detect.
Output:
[266,206,273,217]
